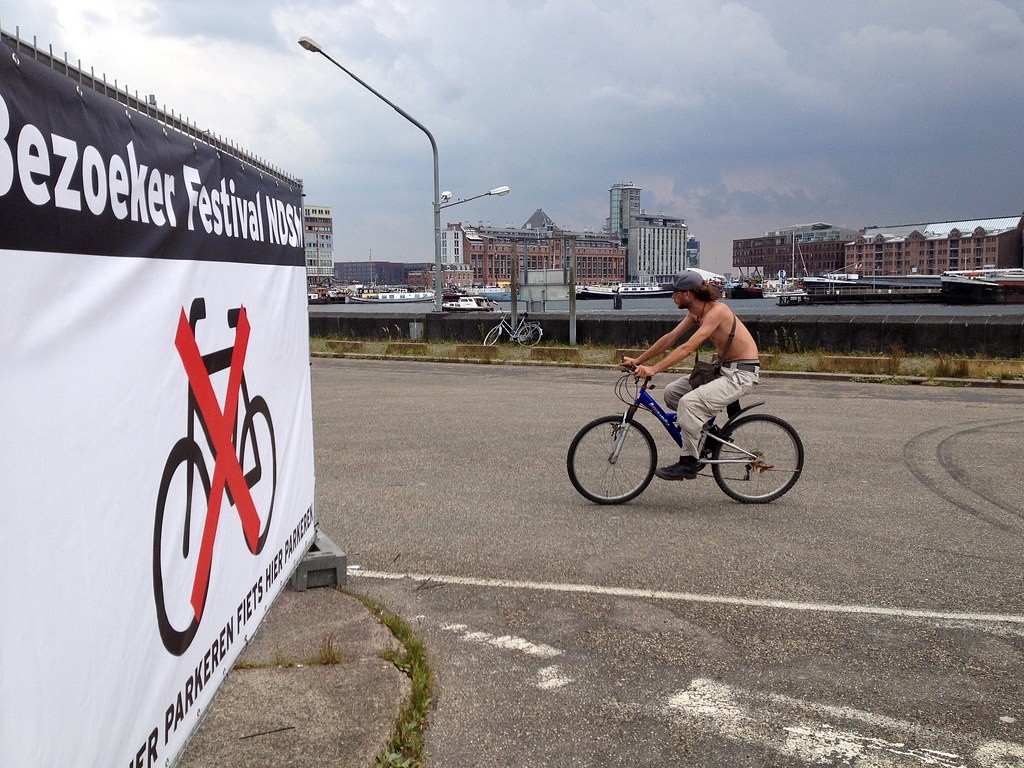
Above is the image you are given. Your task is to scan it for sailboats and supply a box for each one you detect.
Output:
[762,230,808,298]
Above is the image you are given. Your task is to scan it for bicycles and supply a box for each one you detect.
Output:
[483,306,543,347]
[567,354,805,505]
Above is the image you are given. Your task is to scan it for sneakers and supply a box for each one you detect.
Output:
[655,456,697,479]
[700,424,722,454]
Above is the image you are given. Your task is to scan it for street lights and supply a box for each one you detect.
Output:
[296,35,511,310]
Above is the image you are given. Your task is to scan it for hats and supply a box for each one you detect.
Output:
[662,270,706,292]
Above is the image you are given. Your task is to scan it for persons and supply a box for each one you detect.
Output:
[618,270,759,481]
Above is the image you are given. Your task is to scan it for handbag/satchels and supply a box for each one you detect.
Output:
[689,360,721,389]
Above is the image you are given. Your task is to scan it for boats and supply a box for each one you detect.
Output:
[942,268,1024,303]
[442,284,469,301]
[723,285,763,298]
[802,273,943,291]
[939,273,999,303]
[576,283,674,299]
[308,287,443,303]
[442,297,494,312]
